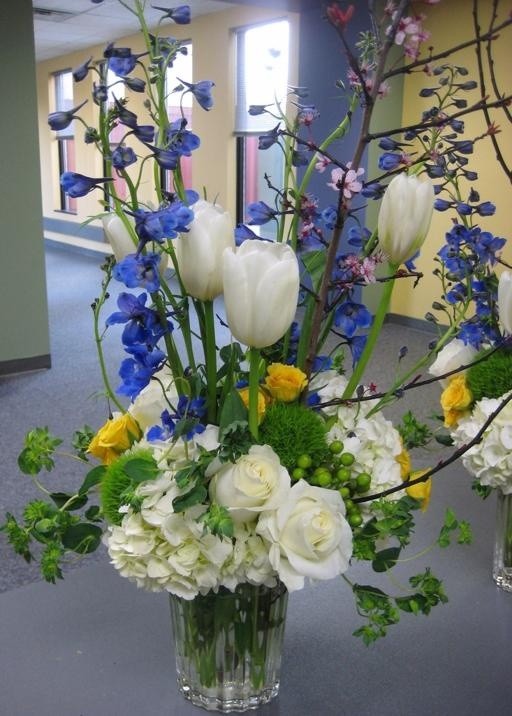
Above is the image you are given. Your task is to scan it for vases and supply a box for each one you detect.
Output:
[161,577,289,716]
